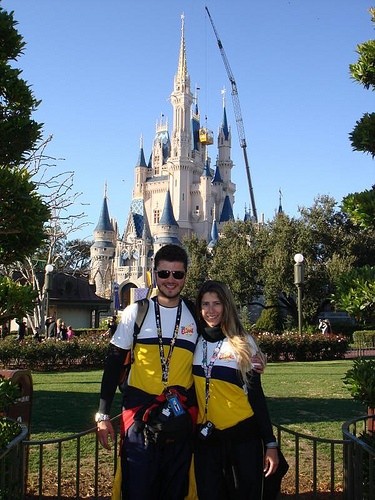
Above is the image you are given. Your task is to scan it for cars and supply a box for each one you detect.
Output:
[312,298,355,326]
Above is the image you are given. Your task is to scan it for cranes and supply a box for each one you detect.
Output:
[204,6,259,225]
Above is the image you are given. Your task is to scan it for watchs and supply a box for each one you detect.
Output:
[95,413,110,422]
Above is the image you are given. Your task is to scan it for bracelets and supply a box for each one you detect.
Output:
[266,442,277,448]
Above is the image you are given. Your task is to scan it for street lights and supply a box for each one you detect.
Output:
[294,253,305,334]
[44,265,54,339]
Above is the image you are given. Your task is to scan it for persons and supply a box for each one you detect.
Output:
[32,327,40,338]
[319,319,332,334]
[57,319,74,340]
[15,317,25,337]
[107,316,117,336]
[45,317,57,339]
[191,280,279,500]
[93,245,264,500]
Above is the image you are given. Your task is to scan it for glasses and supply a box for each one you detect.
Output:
[155,270,186,280]
